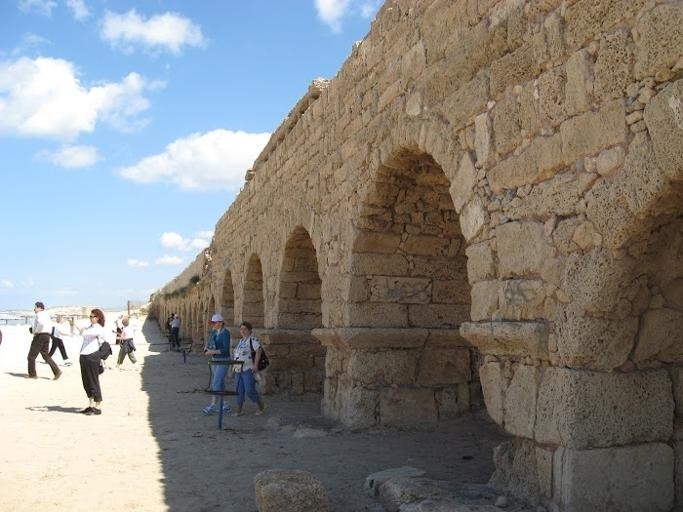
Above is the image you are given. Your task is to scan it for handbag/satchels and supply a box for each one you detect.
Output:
[97,340,112,361]
[246,336,269,371]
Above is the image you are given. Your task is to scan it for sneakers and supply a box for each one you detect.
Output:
[78,406,101,415]
[199,400,231,416]
[231,406,266,417]
[22,370,63,381]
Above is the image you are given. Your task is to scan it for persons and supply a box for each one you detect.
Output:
[108,318,140,370]
[230,320,269,417]
[26,301,63,381]
[164,313,174,343]
[40,313,73,366]
[203,313,232,416]
[114,314,125,345]
[67,309,104,416]
[168,314,182,348]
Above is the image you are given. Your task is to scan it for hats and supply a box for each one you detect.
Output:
[207,313,224,324]
